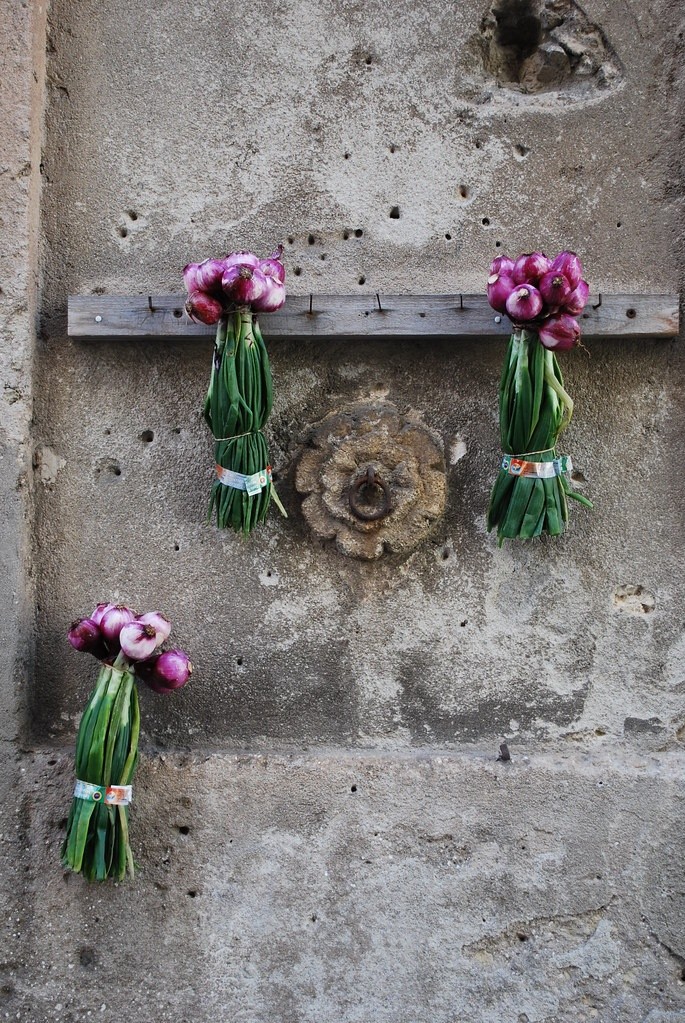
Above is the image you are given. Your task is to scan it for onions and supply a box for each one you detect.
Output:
[67,601,193,694]
[182,251,287,325]
[487,249,589,351]
